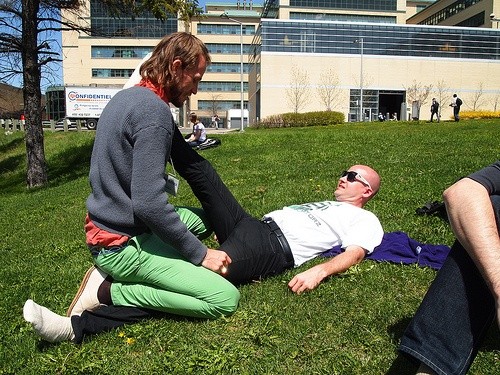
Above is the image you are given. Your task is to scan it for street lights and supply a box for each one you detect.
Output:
[353,38,364,122]
[220,13,243,132]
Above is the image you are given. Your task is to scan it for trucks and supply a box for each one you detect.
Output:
[65,86,179,131]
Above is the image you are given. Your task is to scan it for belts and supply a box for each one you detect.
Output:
[263,217,295,269]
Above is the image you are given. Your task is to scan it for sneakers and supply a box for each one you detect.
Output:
[67,265,107,316]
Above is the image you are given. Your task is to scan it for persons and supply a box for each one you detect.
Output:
[430,98,439,123]
[185,113,207,147]
[386,163,500,375]
[213,115,219,130]
[450,94,460,121]
[67,31,240,321]
[377,113,384,122]
[389,114,397,121]
[22,113,384,344]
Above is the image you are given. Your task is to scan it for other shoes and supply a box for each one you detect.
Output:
[121,52,153,90]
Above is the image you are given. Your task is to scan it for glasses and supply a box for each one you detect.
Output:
[342,171,372,189]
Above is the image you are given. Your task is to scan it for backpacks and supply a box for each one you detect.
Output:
[456,98,462,106]
[197,137,221,150]
